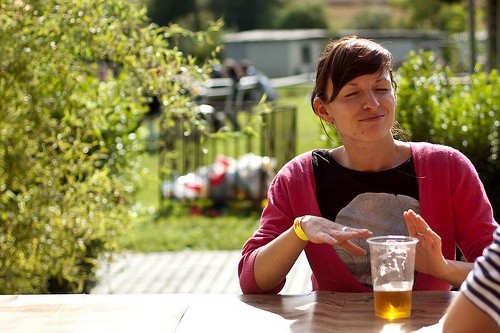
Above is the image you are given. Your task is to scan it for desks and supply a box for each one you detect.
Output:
[0,290,459,333]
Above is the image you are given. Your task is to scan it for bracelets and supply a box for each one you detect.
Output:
[293,216,308,243]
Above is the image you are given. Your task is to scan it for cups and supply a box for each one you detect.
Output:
[366,235,419,320]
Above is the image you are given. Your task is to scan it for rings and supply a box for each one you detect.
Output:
[417,231,422,236]
[343,225,348,231]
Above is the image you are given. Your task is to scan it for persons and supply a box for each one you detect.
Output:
[441,227,500,333]
[237,34,499,294]
